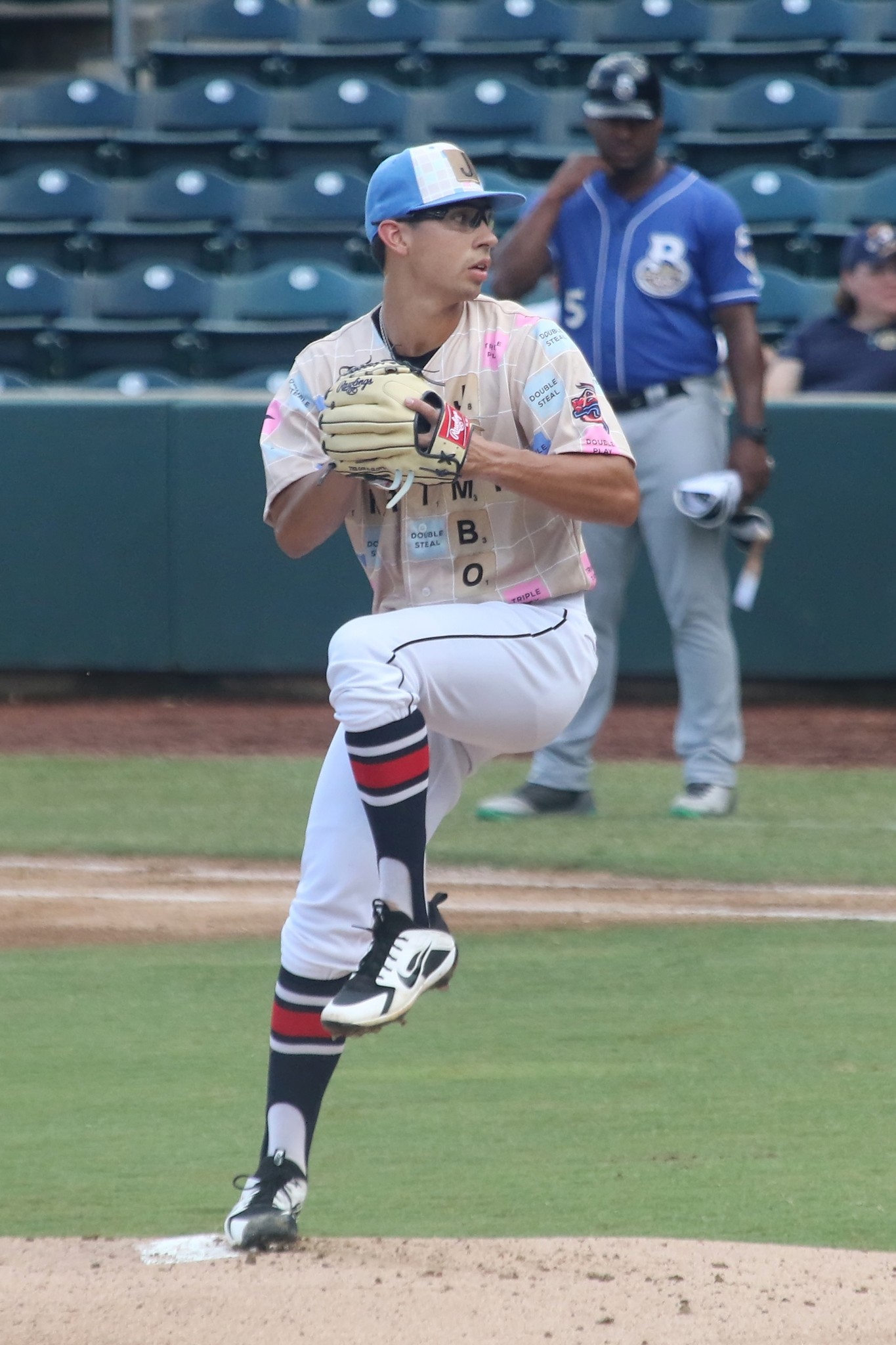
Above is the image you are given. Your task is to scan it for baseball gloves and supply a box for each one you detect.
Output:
[316,359,476,487]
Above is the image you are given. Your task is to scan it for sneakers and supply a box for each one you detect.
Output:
[670,785,736,818]
[472,782,595,824]
[226,1147,312,1253]
[321,893,458,1040]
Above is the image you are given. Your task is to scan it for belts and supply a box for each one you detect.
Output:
[609,381,685,414]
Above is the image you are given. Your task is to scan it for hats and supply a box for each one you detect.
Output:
[584,52,662,121]
[841,224,896,270]
[364,140,526,244]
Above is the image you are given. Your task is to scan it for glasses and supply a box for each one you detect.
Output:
[371,201,495,234]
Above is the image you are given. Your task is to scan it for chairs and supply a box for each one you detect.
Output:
[0,0,896,393]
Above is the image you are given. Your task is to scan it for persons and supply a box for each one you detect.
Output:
[758,224,896,402]
[472,44,774,824]
[224,136,643,1249]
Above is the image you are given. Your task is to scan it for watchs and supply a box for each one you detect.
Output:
[736,424,770,447]
